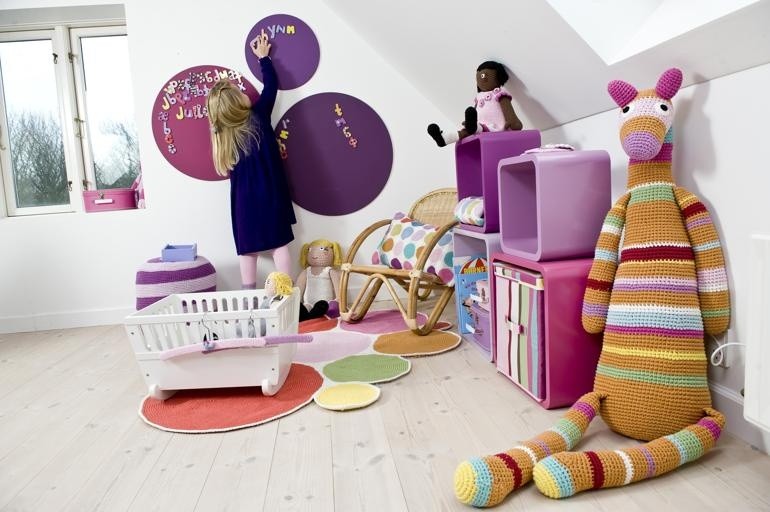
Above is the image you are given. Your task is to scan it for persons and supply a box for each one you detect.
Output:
[205,37,297,309]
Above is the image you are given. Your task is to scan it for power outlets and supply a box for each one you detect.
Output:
[706,328,733,369]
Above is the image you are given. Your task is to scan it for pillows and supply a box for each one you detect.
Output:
[373,210,457,291]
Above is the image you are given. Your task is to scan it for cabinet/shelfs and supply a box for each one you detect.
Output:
[451,128,613,410]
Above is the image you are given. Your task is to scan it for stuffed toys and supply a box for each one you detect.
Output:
[234,271,297,338]
[428,61,522,147]
[453,69,731,508]
[292,238,342,320]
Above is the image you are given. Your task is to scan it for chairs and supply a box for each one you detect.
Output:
[337,186,461,336]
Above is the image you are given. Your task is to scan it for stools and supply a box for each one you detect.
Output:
[135,256,219,311]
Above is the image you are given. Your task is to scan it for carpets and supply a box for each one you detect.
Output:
[138,305,463,434]
